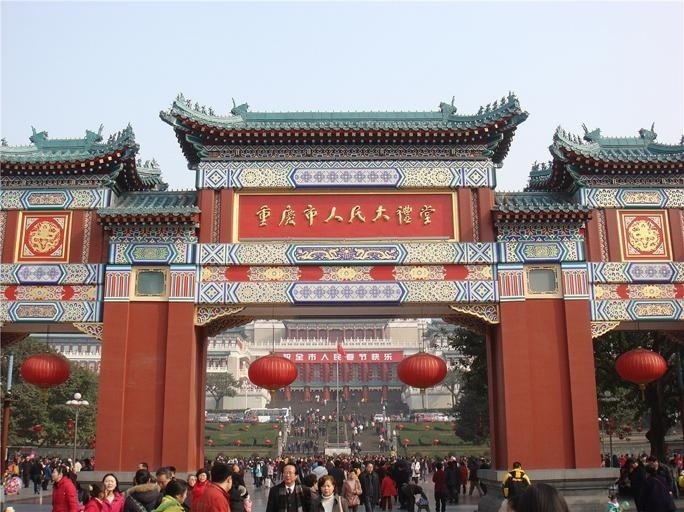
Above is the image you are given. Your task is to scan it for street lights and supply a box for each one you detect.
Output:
[598,414,609,465]
[64,392,92,472]
[241,380,253,410]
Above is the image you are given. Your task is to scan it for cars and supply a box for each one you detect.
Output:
[373,411,453,423]
[205,411,257,424]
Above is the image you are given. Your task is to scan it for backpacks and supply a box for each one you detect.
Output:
[503,471,529,499]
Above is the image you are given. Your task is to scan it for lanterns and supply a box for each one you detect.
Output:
[397,351,447,394]
[20,350,71,395]
[615,347,668,401]
[247,351,299,397]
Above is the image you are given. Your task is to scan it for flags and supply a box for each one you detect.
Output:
[337,344,346,357]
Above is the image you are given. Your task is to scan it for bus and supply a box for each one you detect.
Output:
[243,407,292,424]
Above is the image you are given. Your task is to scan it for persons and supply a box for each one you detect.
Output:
[501,462,532,499]
[84,456,252,512]
[600,450,684,512]
[252,453,490,512]
[206,394,461,453]
[1,447,95,512]
[506,482,568,512]
